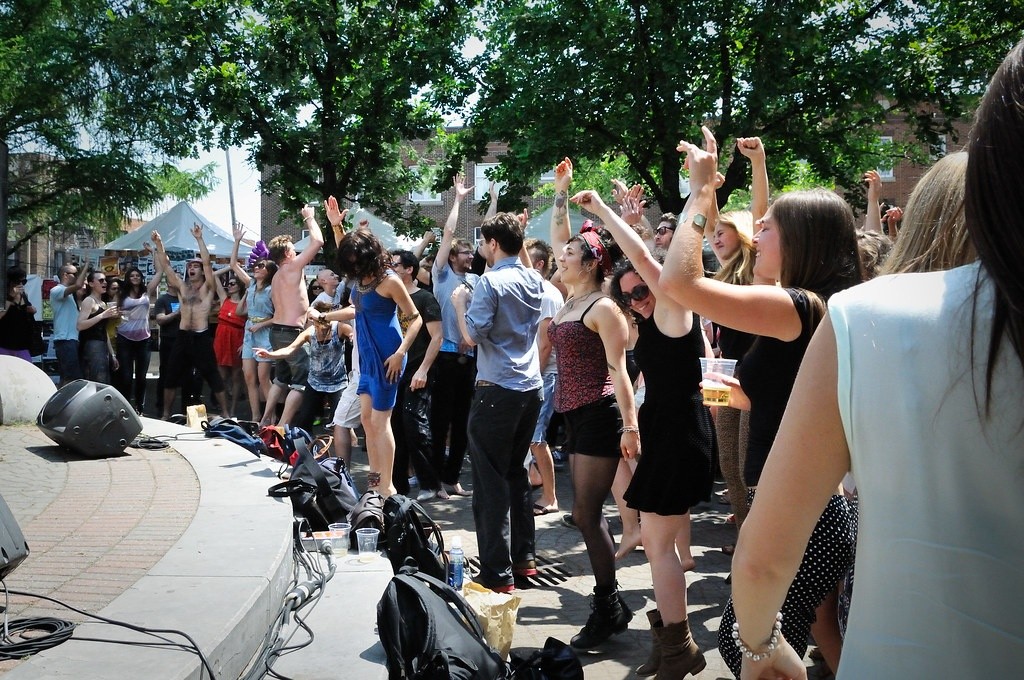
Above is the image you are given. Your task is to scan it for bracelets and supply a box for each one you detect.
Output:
[733,612,784,661]
[620,427,639,435]
[302,215,313,222]
[675,212,707,230]
[332,222,347,240]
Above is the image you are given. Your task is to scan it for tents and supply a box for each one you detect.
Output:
[102,200,260,260]
[292,204,431,269]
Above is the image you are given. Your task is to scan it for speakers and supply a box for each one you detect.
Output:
[0,494,30,581]
[35,379,142,458]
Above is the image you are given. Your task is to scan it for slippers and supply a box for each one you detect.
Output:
[532,502,559,515]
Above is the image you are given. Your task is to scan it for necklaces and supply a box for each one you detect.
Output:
[568,289,600,309]
[226,296,240,316]
[314,328,332,353]
[356,271,386,293]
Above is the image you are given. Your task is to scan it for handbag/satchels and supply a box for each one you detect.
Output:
[28,312,46,357]
[510,637,585,680]
[345,489,387,551]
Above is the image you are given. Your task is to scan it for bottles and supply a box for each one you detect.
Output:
[448,536,464,591]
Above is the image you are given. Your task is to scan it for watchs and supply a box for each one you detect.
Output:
[317,311,327,324]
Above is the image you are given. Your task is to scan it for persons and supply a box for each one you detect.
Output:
[321,196,424,501]
[391,125,979,680]
[306,305,362,471]
[1,204,351,428]
[728,31,1024,680]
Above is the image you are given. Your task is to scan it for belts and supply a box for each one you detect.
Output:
[272,327,296,332]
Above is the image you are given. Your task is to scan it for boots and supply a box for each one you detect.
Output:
[569,579,633,648]
[635,609,707,680]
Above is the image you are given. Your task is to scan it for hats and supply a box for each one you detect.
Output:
[186,257,203,268]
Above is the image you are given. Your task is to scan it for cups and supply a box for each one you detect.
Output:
[355,527,380,563]
[107,302,117,315]
[328,522,352,559]
[699,358,738,407]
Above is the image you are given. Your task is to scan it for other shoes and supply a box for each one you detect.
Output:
[562,513,579,530]
[409,476,420,488]
[551,448,564,467]
[416,489,438,503]
[495,559,537,594]
[721,543,736,555]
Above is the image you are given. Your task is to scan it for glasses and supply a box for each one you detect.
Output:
[227,282,236,286]
[67,271,78,278]
[458,250,473,255]
[14,279,28,286]
[618,283,650,305]
[390,261,403,268]
[254,262,267,269]
[95,278,108,284]
[652,226,673,237]
[311,285,320,291]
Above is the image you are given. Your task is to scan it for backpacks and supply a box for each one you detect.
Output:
[376,556,518,680]
[199,410,334,470]
[382,494,449,584]
[267,437,361,532]
